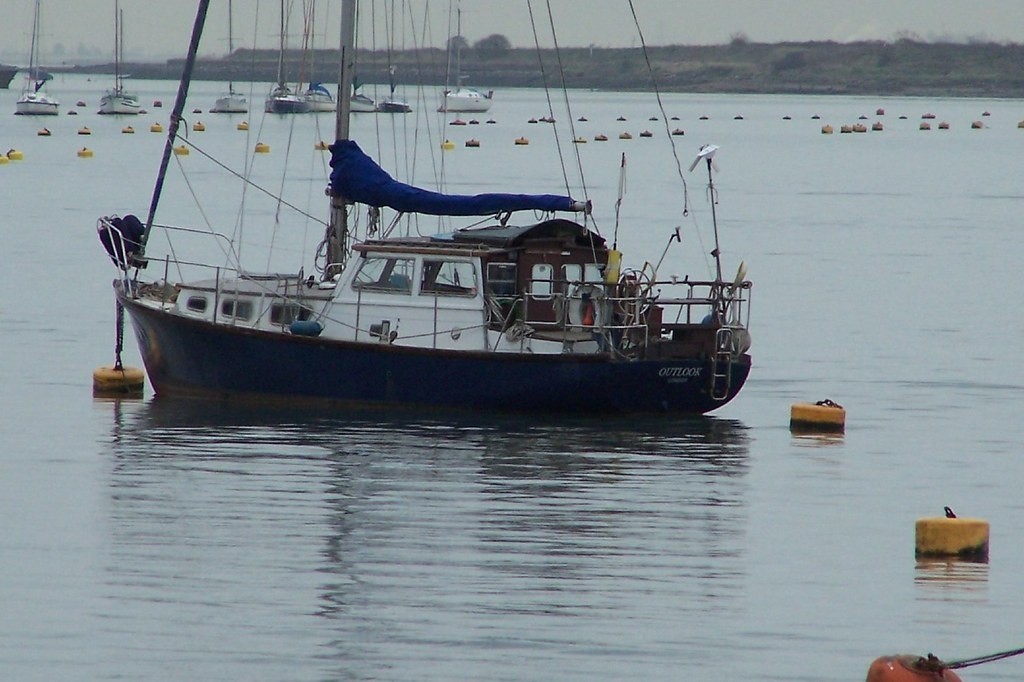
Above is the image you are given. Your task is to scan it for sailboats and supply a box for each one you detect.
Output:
[15,1,60,116]
[98,1,753,418]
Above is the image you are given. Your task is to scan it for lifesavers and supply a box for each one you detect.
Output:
[491,300,521,329]
[569,285,612,334]
